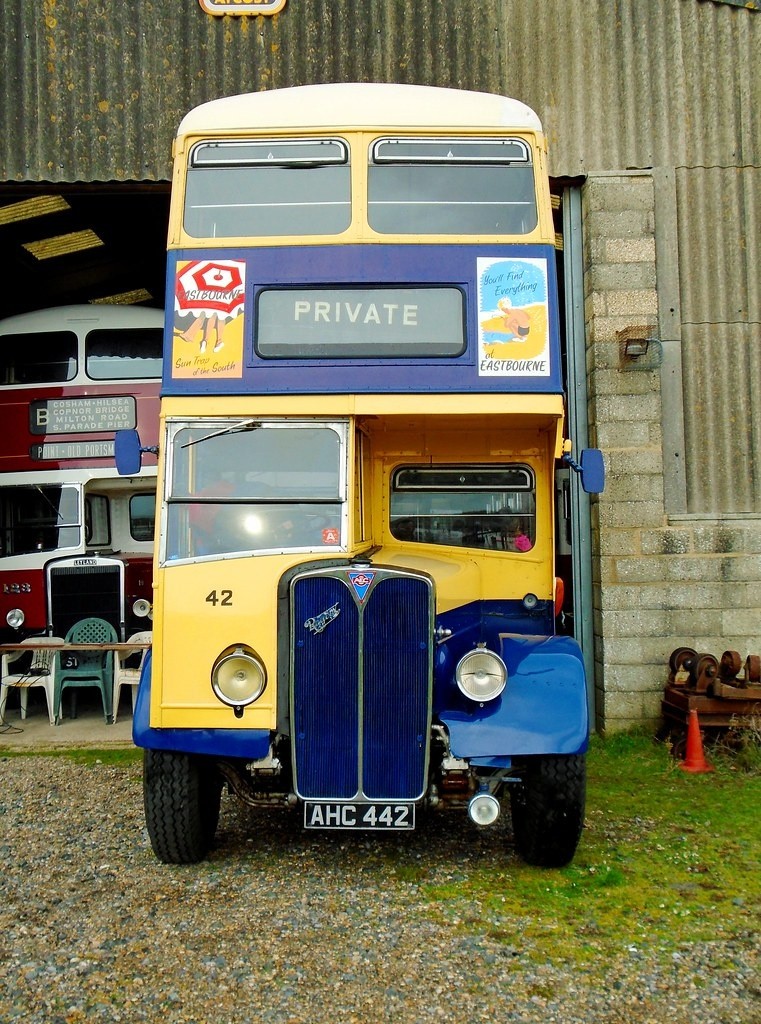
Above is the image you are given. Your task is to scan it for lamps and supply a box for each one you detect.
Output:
[625,338,648,362]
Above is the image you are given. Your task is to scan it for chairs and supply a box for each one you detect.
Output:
[0,619,153,725]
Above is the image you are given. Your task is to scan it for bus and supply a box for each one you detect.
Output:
[112,79,608,869]
[0,304,197,712]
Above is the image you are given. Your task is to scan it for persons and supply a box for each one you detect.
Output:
[387,506,536,551]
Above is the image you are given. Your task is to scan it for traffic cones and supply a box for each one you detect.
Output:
[677,708,717,773]
